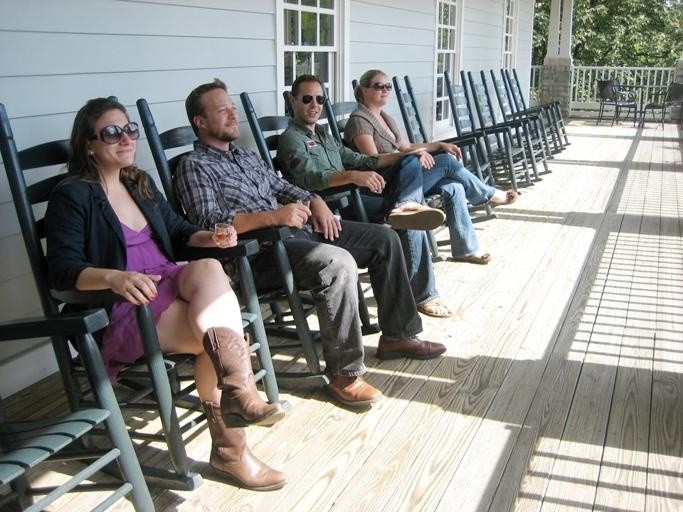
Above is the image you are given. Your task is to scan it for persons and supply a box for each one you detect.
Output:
[275,76,455,322]
[172,75,447,405]
[46,98,285,490]
[344,69,517,264]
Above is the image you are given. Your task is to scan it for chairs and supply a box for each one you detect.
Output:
[137,93,360,376]
[594,78,682,128]
[0,308,158,511]
[1,104,284,491]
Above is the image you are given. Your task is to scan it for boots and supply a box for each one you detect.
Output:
[203,327,285,427]
[201,400,288,490]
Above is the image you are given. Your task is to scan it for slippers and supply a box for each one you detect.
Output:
[454,254,490,264]
[388,205,445,230]
[491,191,517,208]
[418,298,453,317]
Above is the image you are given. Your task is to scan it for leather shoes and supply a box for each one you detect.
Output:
[326,375,383,406]
[376,336,446,359]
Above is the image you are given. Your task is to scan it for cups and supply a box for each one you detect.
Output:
[213,223,233,248]
[296,198,310,209]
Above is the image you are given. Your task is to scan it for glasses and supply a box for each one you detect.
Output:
[295,96,325,104]
[368,83,392,91]
[88,122,139,144]
